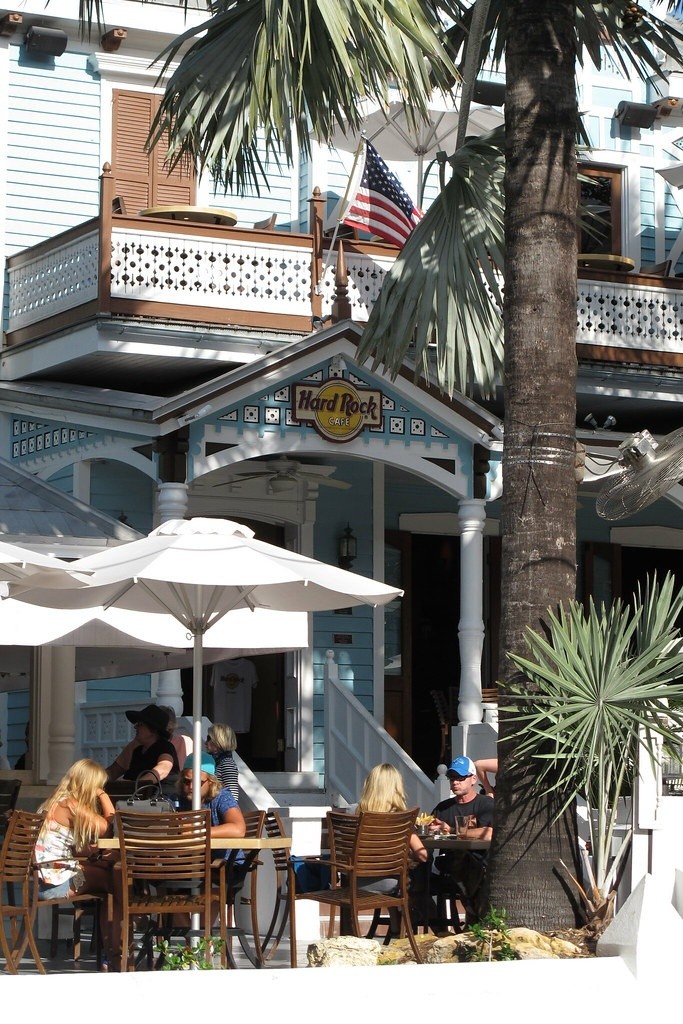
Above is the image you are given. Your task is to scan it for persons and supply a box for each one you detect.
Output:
[430,757,496,908]
[164,751,246,928]
[10,721,29,809]
[474,758,498,799]
[205,722,239,802]
[355,763,441,935]
[28,759,122,971]
[105,705,193,784]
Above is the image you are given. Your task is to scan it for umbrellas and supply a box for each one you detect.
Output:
[308,86,504,206]
[6,518,404,970]
[656,162,683,189]
[0,539,94,578]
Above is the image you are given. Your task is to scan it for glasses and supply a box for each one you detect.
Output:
[184,776,210,787]
[206,735,211,741]
[135,722,145,728]
[448,774,473,781]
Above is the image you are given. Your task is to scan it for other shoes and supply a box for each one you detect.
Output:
[100,956,117,972]
[134,935,168,950]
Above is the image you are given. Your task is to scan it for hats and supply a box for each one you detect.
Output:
[446,757,476,776]
[125,705,172,741]
[183,751,215,776]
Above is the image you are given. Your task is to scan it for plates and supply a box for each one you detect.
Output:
[417,835,429,839]
[432,833,457,838]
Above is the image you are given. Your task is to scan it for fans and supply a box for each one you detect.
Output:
[210,455,353,493]
[577,427,683,521]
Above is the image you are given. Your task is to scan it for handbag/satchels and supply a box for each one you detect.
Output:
[113,770,180,842]
[286,852,340,894]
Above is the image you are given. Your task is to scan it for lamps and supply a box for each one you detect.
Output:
[340,520,357,568]
[270,476,296,489]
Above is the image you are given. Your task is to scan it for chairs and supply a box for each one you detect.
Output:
[324,225,359,240]
[114,196,126,213]
[0,779,266,974]
[254,214,277,230]
[261,809,424,968]
[639,260,672,276]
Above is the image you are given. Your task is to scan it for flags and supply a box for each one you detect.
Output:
[336,135,421,248]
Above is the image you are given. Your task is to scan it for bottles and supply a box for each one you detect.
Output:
[173,796,180,812]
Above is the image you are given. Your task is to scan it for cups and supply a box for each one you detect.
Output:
[455,815,468,840]
[415,825,428,835]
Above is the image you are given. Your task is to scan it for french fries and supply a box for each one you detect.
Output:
[415,812,434,826]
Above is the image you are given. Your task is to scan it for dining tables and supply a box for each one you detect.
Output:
[419,839,491,849]
[99,837,292,970]
[579,253,635,271]
[139,206,237,226]
